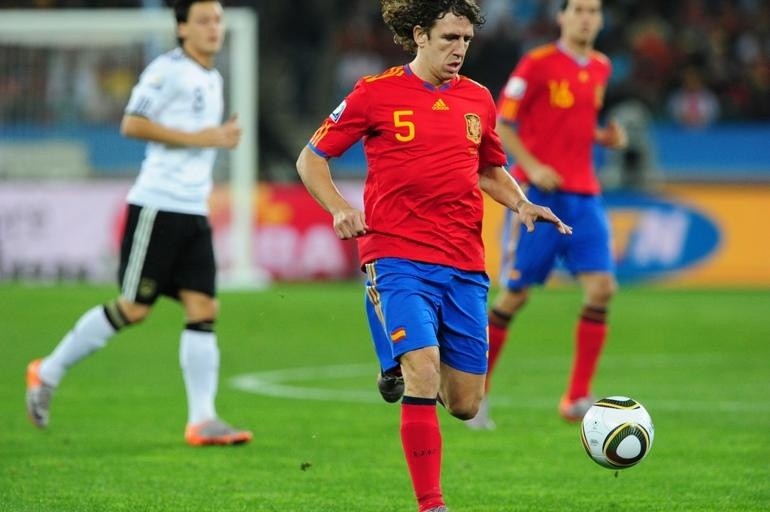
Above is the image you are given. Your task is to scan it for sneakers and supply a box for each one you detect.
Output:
[559,393,599,422]
[185,416,253,446]
[376,362,405,403]
[465,395,497,431]
[25,359,58,429]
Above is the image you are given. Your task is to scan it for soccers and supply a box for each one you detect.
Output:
[581,395,655,470]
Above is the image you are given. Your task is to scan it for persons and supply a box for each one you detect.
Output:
[467,0,617,432]
[255,1,560,185]
[294,0,576,512]
[22,0,255,450]
[592,1,769,185]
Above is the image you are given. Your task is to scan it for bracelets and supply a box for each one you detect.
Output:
[515,200,531,214]
